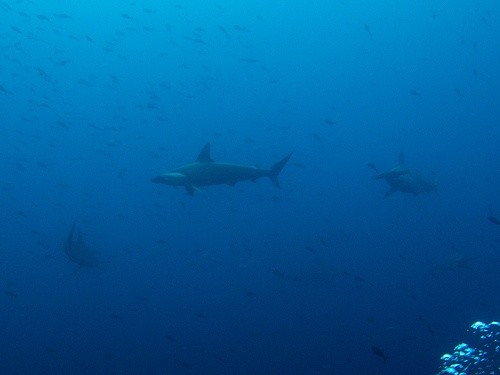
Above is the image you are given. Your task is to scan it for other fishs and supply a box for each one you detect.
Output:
[0,0,500,375]
